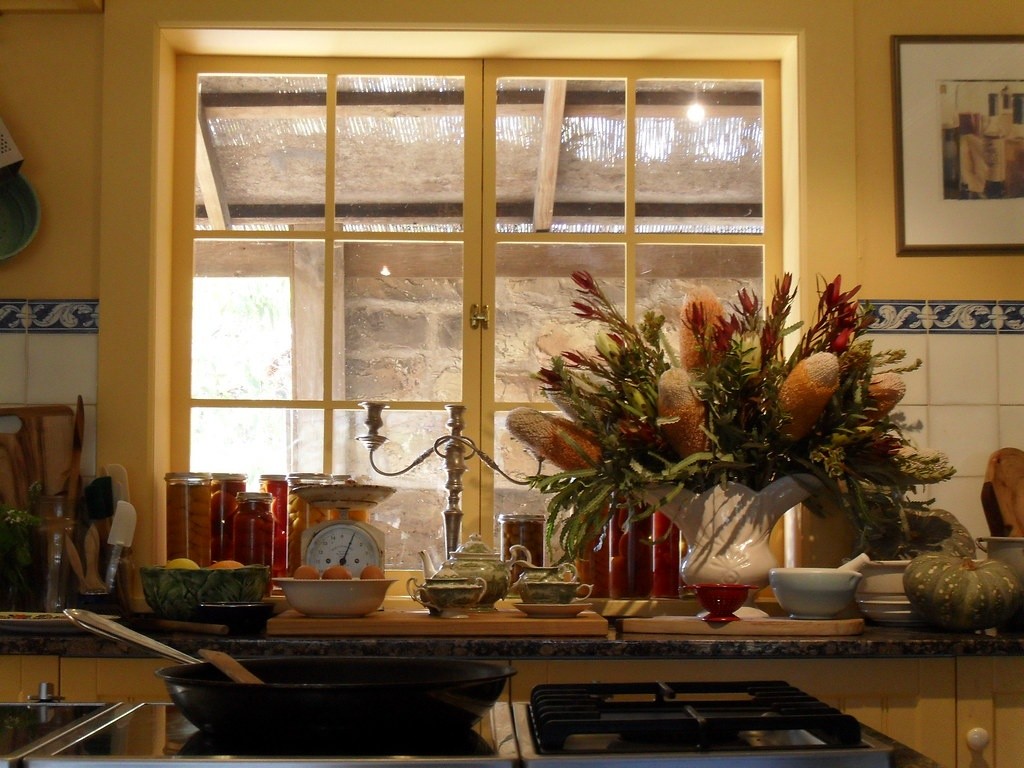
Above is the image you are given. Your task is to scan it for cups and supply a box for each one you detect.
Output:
[404,574,487,618]
[512,558,593,604]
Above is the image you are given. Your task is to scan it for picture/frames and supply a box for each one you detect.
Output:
[892,35,1024,256]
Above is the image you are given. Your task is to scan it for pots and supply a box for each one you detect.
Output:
[64,605,520,746]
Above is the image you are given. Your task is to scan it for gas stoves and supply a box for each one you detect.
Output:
[12,671,945,767]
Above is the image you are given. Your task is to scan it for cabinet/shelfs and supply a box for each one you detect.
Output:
[0,609,1023,768]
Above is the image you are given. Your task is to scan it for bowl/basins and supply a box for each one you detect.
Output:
[271,570,400,618]
[140,559,270,627]
[686,582,753,621]
[767,566,863,621]
[194,596,281,639]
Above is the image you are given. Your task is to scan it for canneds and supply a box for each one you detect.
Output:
[499,512,546,591]
[574,486,692,600]
[165,471,368,579]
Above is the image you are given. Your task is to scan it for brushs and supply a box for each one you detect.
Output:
[65,523,110,616]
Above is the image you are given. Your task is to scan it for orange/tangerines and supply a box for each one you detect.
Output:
[362,566,386,579]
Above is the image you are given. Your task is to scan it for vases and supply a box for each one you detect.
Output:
[629,474,823,622]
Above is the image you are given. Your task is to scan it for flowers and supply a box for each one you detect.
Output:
[525,268,959,546]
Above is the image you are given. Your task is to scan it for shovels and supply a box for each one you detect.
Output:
[104,500,137,594]
[83,475,129,614]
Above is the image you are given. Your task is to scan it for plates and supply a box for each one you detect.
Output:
[0,608,121,633]
[513,600,593,618]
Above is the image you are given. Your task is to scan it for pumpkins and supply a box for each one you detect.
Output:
[902,551,1021,630]
[890,501,978,561]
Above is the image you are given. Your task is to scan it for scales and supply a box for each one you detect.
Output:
[289,482,399,580]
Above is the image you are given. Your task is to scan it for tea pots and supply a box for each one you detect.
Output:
[418,531,532,612]
